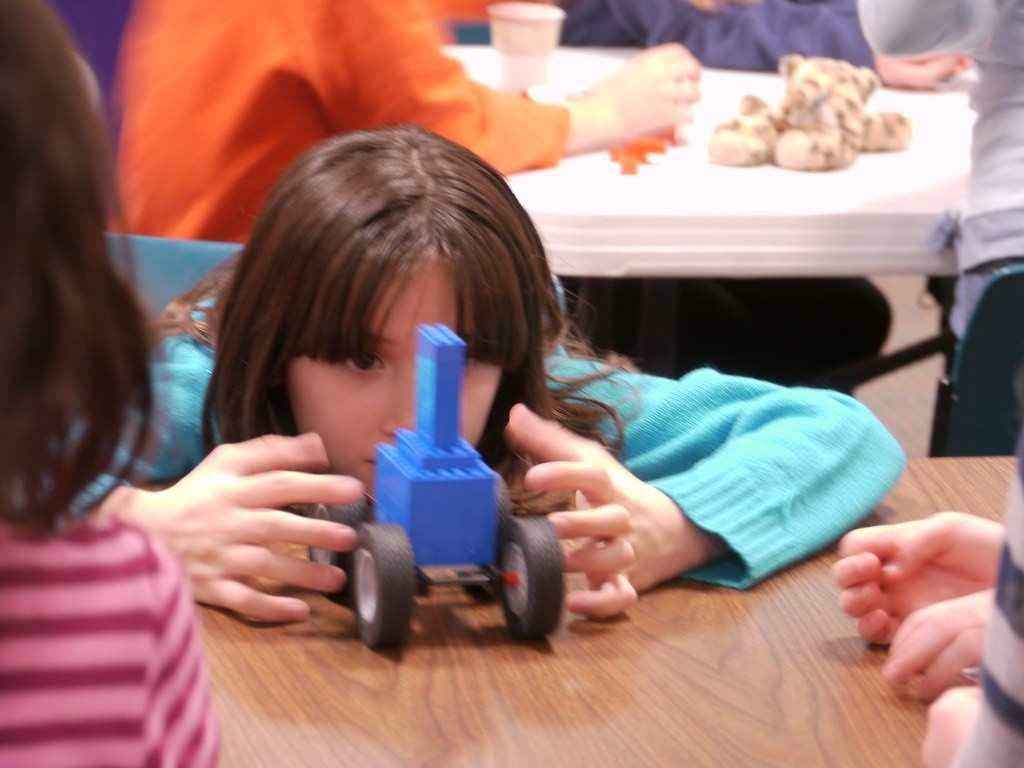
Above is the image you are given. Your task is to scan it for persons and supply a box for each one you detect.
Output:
[565,0,893,392]
[105,0,701,242]
[833,430,1023,767]
[59,127,907,622]
[855,0,1024,462]
[1,1,219,767]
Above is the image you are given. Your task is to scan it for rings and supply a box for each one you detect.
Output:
[964,667,980,678]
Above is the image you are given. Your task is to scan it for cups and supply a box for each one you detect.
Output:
[487,3,565,98]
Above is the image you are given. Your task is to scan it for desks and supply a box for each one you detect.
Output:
[442,43,977,388]
[131,455,1018,768]
